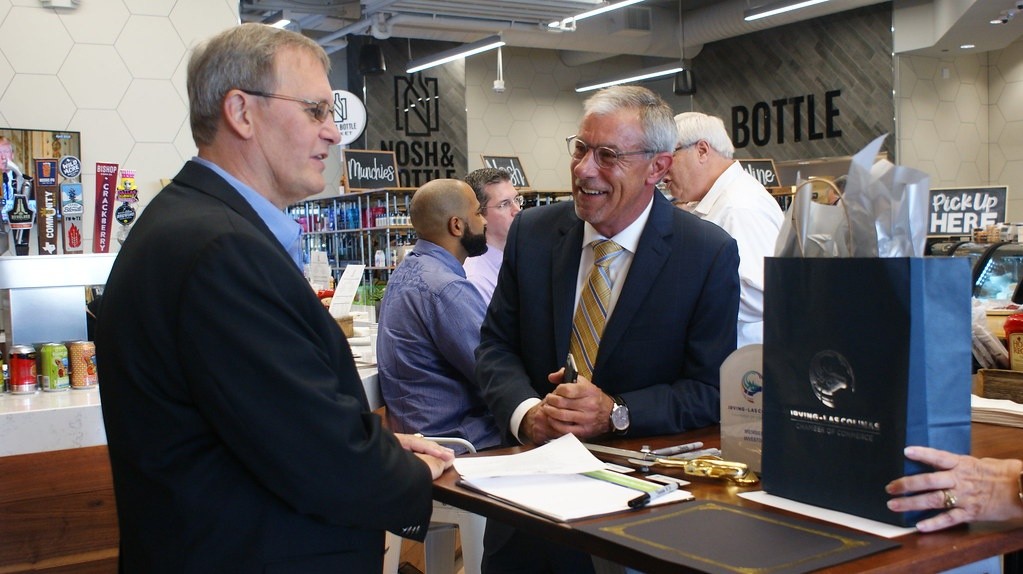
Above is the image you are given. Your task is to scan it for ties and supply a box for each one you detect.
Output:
[568,240,626,383]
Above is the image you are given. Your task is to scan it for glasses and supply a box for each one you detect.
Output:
[482,196,523,211]
[566,135,657,169]
[241,88,335,123]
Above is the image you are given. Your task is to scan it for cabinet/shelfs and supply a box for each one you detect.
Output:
[285,186,572,305]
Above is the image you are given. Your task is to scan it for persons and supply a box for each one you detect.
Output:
[379,178,518,453]
[475,85,739,574]
[662,111,785,349]
[462,168,524,308]
[96,23,454,573]
[886,446,1023,533]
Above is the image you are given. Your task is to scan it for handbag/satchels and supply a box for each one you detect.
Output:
[758,178,972,529]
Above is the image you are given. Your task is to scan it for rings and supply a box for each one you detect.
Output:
[943,489,956,509]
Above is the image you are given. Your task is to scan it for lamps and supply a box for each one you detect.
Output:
[545,0,644,31]
[405,33,506,93]
[261,9,291,28]
[744,0,829,21]
[574,0,686,92]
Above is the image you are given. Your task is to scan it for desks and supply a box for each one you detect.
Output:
[427,374,1023,573]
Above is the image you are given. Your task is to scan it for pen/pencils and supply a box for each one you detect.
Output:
[628,481,683,508]
[651,442,704,457]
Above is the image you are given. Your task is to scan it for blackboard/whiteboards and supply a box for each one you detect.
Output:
[342,148,400,192]
[926,189,1009,238]
[480,154,531,190]
[738,156,780,191]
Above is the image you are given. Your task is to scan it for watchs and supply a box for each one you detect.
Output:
[610,394,630,435]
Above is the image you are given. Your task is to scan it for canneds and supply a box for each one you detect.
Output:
[9,344,37,394]
[40,343,70,392]
[69,341,97,388]
[0,352,5,396]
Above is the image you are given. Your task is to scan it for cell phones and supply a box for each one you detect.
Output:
[561,354,579,384]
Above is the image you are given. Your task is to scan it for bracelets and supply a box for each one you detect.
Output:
[1019,472,1023,504]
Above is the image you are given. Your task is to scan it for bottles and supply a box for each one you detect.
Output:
[316,202,411,230]
[1004,311,1023,373]
